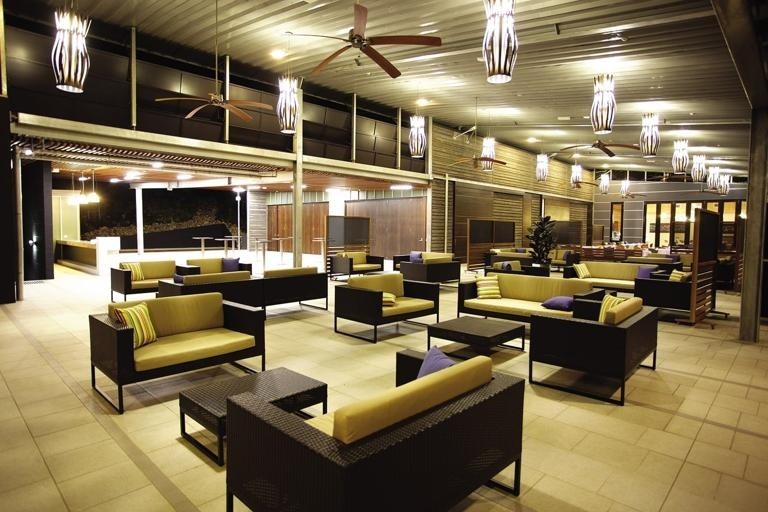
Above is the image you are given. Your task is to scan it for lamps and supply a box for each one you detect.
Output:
[619,180,633,199]
[670,138,691,173]
[570,145,582,190]
[706,167,720,192]
[590,74,616,136]
[690,155,710,182]
[271,31,303,136]
[719,175,732,196]
[599,174,611,195]
[535,136,549,183]
[47,0,95,99]
[640,112,661,163]
[480,0,520,86]
[165,180,174,191]
[407,89,430,160]
[63,167,102,206]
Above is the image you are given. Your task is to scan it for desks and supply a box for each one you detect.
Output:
[427,315,525,361]
[178,366,328,467]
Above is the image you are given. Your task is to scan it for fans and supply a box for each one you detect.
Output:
[440,94,505,171]
[151,0,277,130]
[284,0,443,83]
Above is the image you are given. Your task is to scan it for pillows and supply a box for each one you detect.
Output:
[112,302,159,349]
[221,257,240,271]
[382,290,397,306]
[173,273,183,285]
[474,275,503,300]
[598,294,627,322]
[409,251,423,263]
[637,265,656,279]
[540,296,574,312]
[668,269,692,282]
[572,263,591,279]
[120,262,144,281]
[416,344,456,381]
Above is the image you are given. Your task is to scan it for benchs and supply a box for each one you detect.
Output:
[329,252,385,282]
[225,349,525,512]
[111,258,252,302]
[563,261,666,292]
[484,260,550,277]
[482,234,694,271]
[155,267,328,320]
[529,297,659,406]
[399,257,460,284]
[633,272,692,312]
[87,292,266,414]
[457,271,616,318]
[334,272,440,344]
[393,251,461,270]
